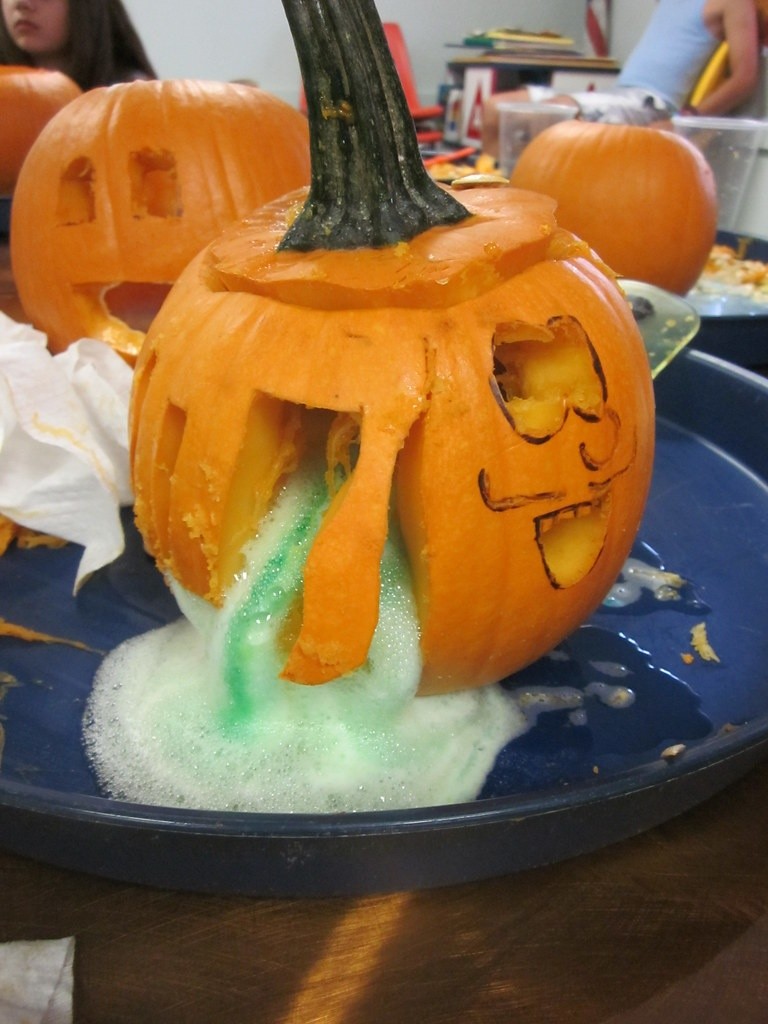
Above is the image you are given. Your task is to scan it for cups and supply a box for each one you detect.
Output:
[498,102,580,179]
[672,116,768,231]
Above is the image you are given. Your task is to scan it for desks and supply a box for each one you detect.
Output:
[450,55,622,150]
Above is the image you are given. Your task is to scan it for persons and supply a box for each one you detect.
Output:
[0,1,157,92]
[481,0,761,170]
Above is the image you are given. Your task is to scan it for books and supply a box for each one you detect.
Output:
[484,25,581,60]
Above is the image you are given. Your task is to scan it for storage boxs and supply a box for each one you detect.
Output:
[497,100,768,229]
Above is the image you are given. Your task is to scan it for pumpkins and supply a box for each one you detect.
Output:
[0,65,716,702]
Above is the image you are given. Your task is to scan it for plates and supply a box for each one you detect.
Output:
[0,348,768,898]
[693,230,768,364]
